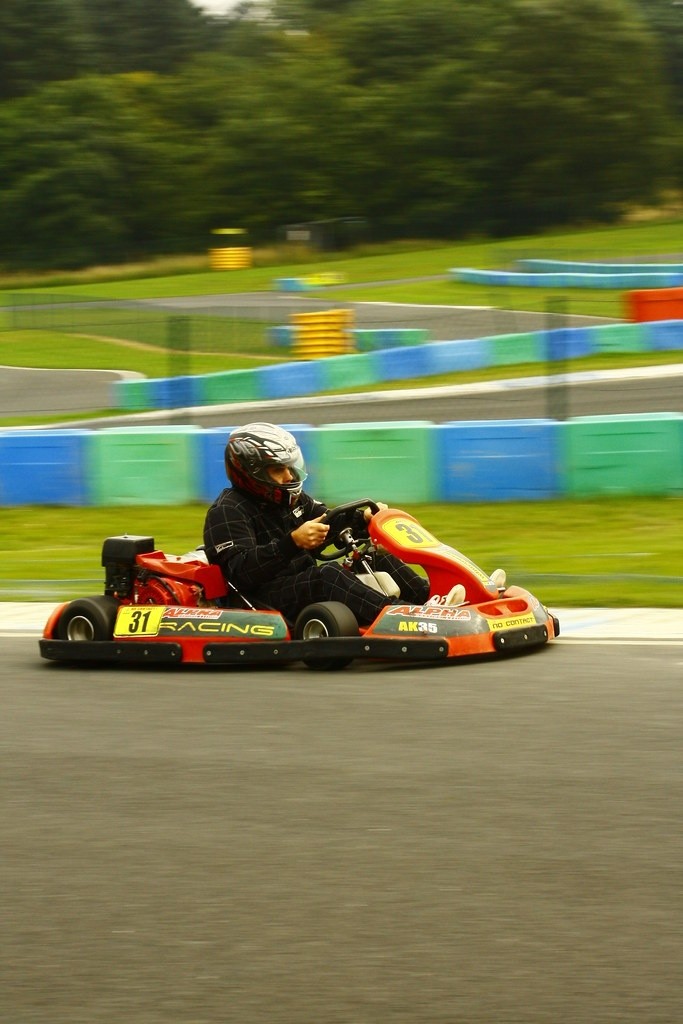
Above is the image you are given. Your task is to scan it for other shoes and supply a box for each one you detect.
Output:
[424,583,465,606]
[489,568,506,588]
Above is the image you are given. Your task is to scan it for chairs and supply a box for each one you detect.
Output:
[135,544,351,636]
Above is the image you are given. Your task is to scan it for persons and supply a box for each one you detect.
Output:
[202,422,508,627]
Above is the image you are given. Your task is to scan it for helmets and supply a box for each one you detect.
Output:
[224,421,309,508]
